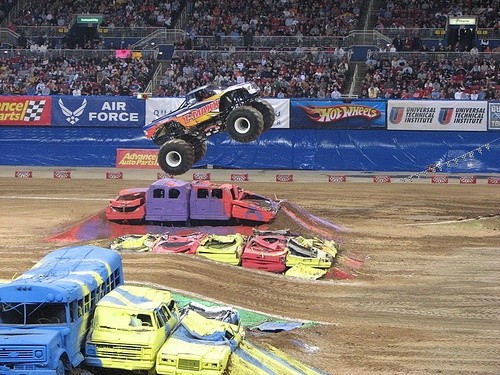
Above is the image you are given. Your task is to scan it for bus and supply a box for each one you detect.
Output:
[0,246,124,375]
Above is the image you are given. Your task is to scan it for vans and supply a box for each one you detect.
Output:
[156,301,245,375]
[109,235,339,280]
[85,285,180,375]
[106,179,281,227]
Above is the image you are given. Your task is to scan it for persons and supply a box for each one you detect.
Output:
[0,0,500,100]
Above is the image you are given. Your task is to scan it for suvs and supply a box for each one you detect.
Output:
[142,82,275,176]
